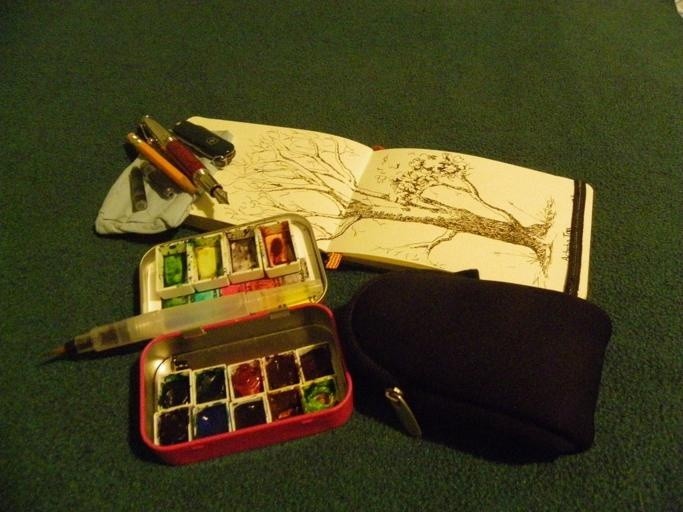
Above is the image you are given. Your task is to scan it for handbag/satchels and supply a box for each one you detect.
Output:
[332,268,611,462]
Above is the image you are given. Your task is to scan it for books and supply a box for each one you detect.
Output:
[184,117,594,300]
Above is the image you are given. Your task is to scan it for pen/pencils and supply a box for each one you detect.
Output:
[127,132,203,202]
[139,114,232,205]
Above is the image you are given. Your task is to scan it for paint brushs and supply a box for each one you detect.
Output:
[41,276,325,366]
